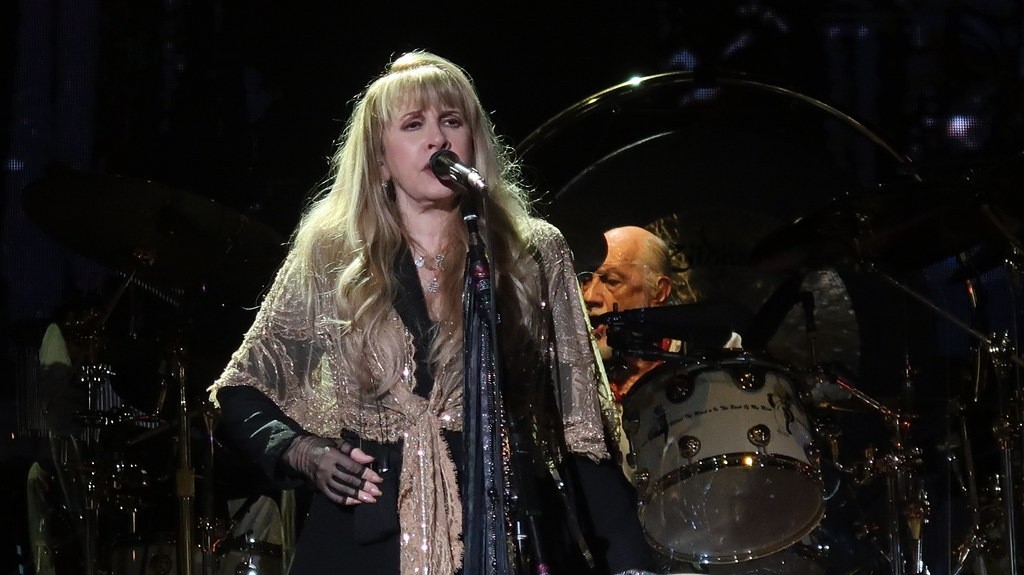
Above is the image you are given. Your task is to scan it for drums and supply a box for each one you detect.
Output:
[216,536,295,575]
[102,530,203,575]
[619,347,827,566]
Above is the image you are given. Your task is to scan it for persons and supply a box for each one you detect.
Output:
[215,48,649,575]
[580,225,679,384]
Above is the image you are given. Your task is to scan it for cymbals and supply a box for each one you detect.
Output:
[746,171,1008,276]
[20,172,286,289]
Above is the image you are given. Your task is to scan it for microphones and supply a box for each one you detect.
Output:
[429,150,488,193]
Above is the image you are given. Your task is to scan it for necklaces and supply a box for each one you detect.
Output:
[412,238,466,296]
